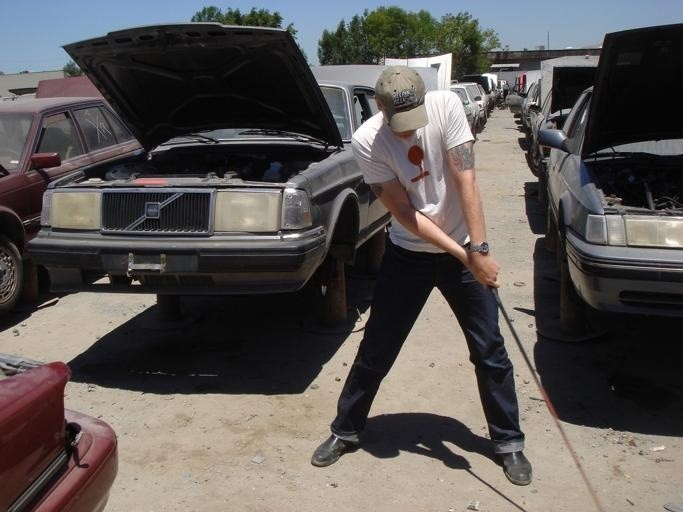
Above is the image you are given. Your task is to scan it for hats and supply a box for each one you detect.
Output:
[376,62,430,133]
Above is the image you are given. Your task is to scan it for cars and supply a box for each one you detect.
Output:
[536,21,683,318]
[27,22,438,328]
[0,76,145,321]
[384,53,683,176]
[0,352,118,512]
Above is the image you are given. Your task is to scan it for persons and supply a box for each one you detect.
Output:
[310,64,533,486]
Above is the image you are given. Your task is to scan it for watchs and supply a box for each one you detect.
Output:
[468,241,489,256]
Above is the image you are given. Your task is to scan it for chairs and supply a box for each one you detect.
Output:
[20,126,68,164]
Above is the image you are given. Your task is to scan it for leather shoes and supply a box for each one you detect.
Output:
[309,433,359,467]
[500,451,534,486]
